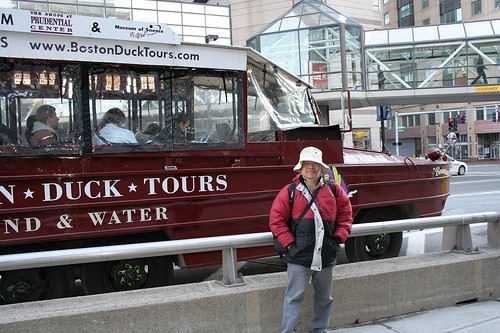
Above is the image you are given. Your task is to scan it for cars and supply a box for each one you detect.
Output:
[417,152,468,176]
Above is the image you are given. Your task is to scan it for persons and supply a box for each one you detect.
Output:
[471,55,488,85]
[378,71,385,89]
[0,105,195,148]
[270,147,353,333]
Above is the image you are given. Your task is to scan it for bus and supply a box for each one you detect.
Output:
[0,6,452,305]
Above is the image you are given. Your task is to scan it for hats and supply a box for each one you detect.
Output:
[293,146,330,172]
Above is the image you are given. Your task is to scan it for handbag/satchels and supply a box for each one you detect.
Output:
[272,233,288,258]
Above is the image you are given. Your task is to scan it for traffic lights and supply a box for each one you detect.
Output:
[492,112,496,122]
[448,117,457,132]
[498,112,500,122]
[457,113,467,124]
